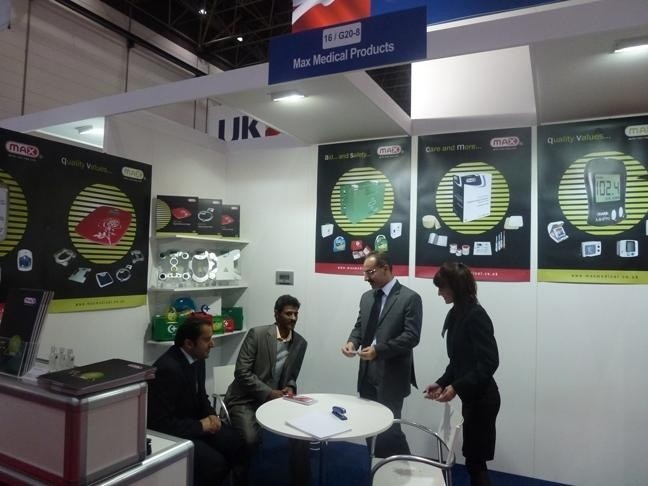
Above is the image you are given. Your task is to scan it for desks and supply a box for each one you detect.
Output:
[254,393,395,486]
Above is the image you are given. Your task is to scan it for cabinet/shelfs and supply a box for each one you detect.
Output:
[0,357,195,485]
[145,233,248,346]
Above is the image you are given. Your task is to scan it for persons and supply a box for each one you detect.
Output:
[423,263,500,486]
[147,317,245,486]
[220,295,307,452]
[341,251,423,457]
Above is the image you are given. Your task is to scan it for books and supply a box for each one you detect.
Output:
[286,411,352,438]
[38,359,156,396]
[1,288,54,375]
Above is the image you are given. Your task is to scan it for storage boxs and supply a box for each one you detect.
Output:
[151,307,243,341]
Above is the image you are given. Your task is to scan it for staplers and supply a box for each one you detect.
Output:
[331,406,348,420]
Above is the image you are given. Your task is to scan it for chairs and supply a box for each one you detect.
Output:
[370,393,465,486]
[211,364,235,426]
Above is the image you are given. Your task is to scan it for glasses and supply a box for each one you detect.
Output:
[362,265,382,276]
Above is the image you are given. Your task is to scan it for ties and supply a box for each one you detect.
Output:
[359,288,384,352]
